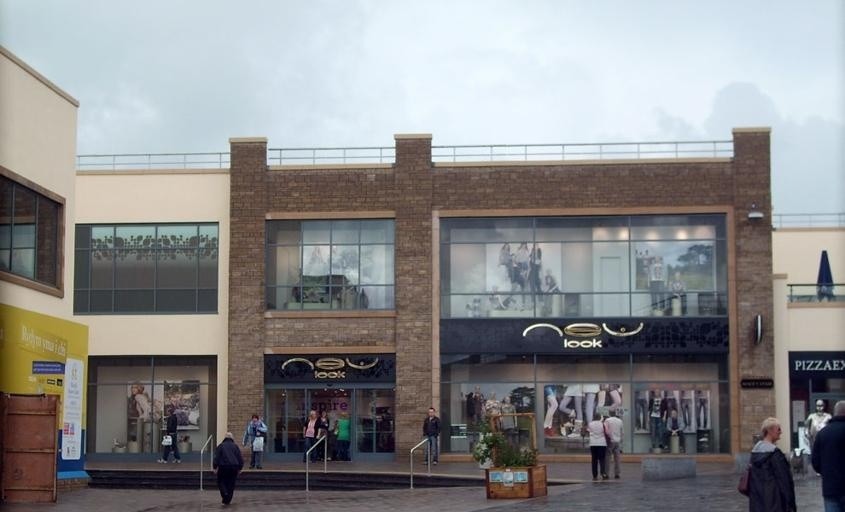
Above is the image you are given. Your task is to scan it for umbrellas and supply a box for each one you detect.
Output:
[817,249,835,301]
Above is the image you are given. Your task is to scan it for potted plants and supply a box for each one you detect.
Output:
[471,420,548,499]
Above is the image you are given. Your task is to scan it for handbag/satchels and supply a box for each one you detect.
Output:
[162,435,172,446]
[738,467,751,497]
[252,436,264,451]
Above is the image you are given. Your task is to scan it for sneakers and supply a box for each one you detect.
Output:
[423,460,437,465]
[158,458,182,464]
[594,474,620,480]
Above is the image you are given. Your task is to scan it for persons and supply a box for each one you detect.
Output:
[469,386,518,446]
[745,417,797,512]
[303,409,352,463]
[489,240,559,313]
[647,255,667,310]
[587,412,611,480]
[213,432,244,506]
[667,270,688,315]
[633,390,708,452]
[130,383,150,419]
[157,406,182,465]
[544,384,622,437]
[812,400,845,511]
[422,408,441,465]
[603,409,624,478]
[242,414,268,469]
[805,399,832,477]
